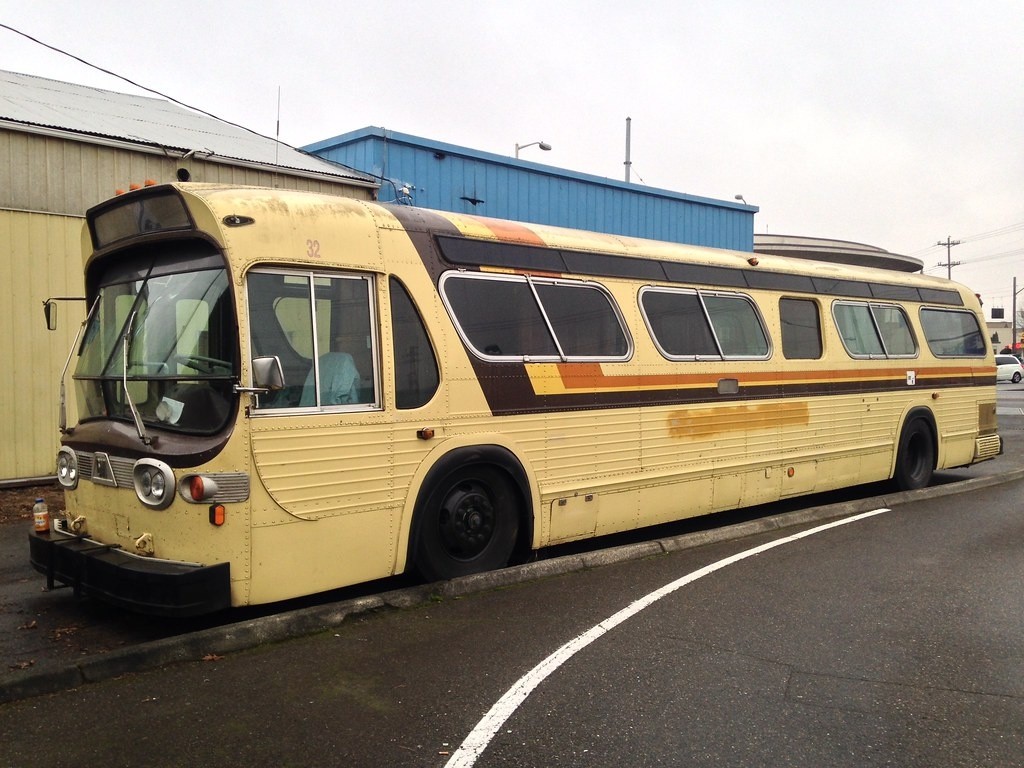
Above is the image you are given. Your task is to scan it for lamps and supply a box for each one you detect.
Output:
[735,194,747,204]
[434,151,445,159]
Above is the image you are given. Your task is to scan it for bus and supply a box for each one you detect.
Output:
[25,180,1003,630]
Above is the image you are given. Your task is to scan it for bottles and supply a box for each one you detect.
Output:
[33,498,50,535]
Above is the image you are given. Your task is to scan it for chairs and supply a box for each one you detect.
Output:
[299,352,359,408]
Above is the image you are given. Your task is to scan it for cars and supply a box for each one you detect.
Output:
[994,353,1024,384]
